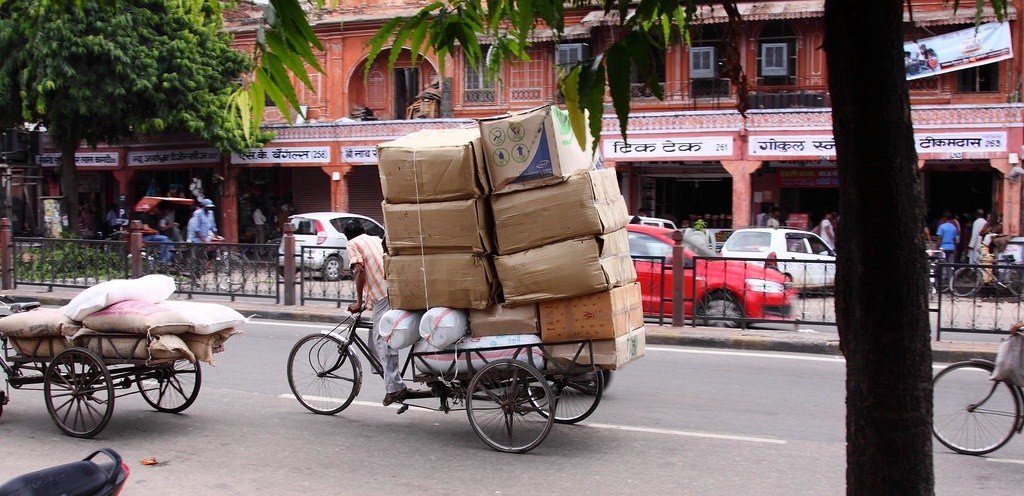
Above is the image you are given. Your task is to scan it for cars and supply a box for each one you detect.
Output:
[625,224,798,329]
[718,228,834,289]
[277,212,387,282]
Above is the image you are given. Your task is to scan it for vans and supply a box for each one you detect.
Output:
[628,216,678,232]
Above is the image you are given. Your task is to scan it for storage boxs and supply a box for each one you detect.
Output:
[375,101,646,370]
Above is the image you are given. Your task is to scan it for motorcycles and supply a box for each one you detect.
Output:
[0,447,130,496]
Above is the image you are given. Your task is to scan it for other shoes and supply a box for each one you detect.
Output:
[383,387,407,406]
[191,281,201,286]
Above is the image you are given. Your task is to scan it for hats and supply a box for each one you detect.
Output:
[201,199,215,208]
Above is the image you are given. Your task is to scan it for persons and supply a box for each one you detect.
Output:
[106,199,225,287]
[343,217,407,406]
[1010,320,1024,335]
[254,204,267,257]
[821,212,835,250]
[924,209,1003,286]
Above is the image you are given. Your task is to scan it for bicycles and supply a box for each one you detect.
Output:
[928,255,952,294]
[948,253,1024,299]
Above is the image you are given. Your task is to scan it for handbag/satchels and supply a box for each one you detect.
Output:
[990,330,1024,388]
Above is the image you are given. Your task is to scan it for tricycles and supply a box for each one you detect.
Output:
[0,326,202,438]
[120,195,249,292]
[932,329,1024,456]
[286,299,603,454]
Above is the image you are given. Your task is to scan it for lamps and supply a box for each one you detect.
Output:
[1008,152,1020,165]
[332,172,340,181]
